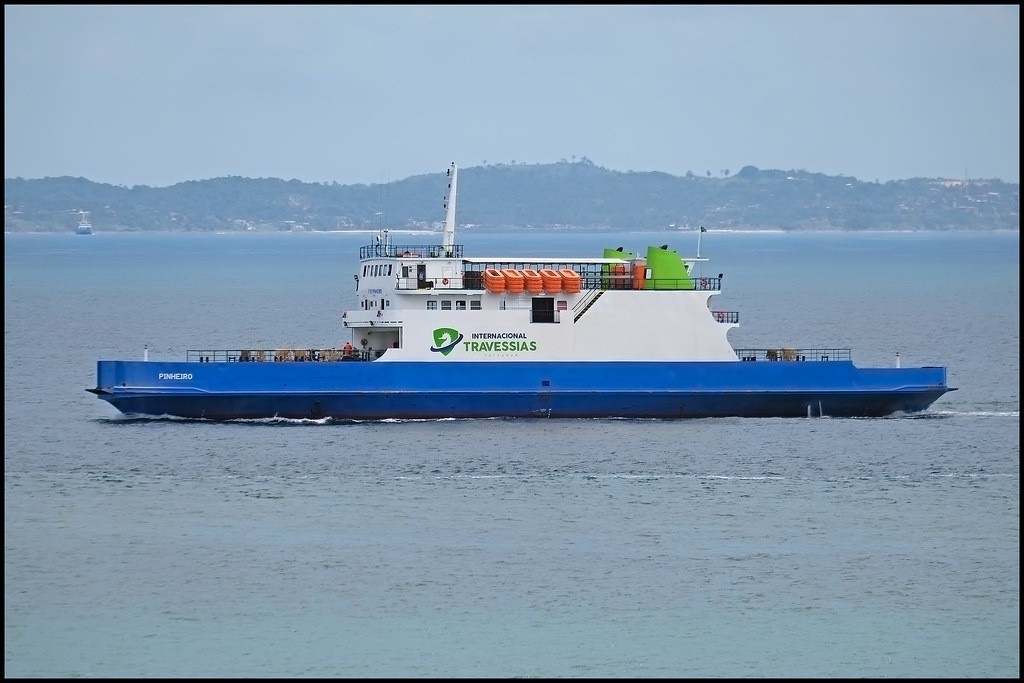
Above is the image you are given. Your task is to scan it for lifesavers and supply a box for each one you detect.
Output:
[442,279,449,285]
[700,279,707,287]
[718,313,725,319]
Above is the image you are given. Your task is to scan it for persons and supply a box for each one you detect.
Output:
[344,342,353,356]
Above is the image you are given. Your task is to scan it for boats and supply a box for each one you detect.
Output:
[78,214,93,234]
[85,160,959,419]
